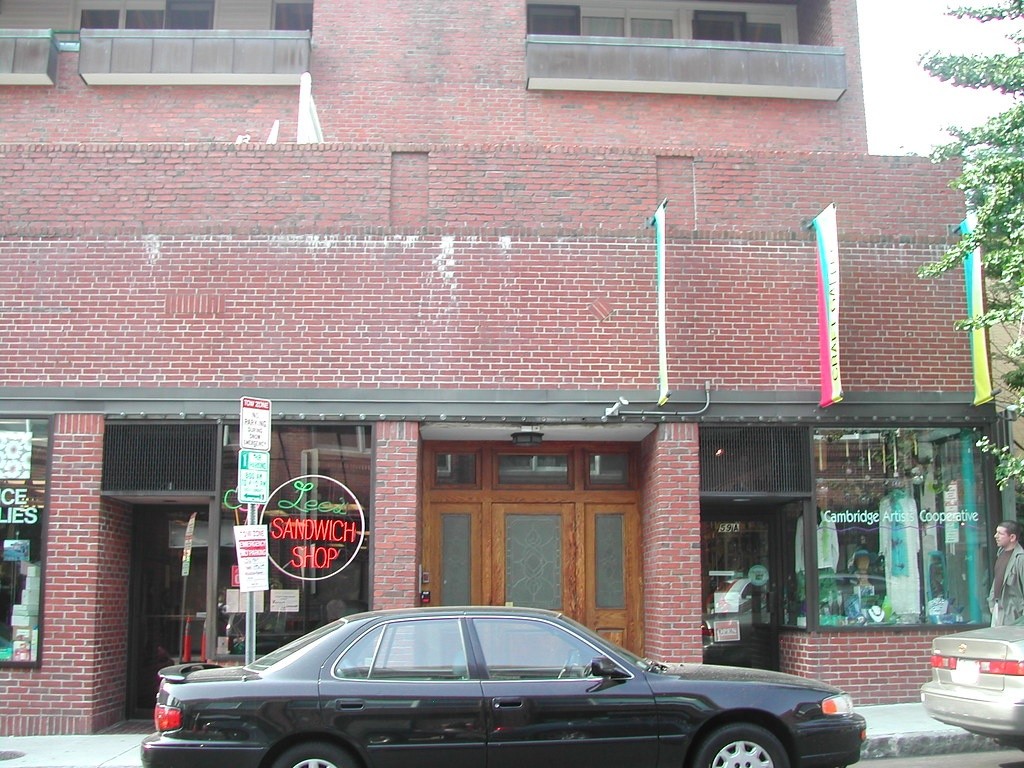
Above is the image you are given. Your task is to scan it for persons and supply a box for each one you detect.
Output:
[794,505,839,603]
[878,487,922,614]
[316,599,346,628]
[988,520,1024,625]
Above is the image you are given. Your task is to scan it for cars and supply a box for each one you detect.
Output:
[142,606,870,768]
[920,626,1024,738]
[814,571,891,619]
[147,602,328,667]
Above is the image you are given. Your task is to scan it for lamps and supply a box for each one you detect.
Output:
[511,432,544,446]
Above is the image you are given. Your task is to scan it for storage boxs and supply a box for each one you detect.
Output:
[9,564,38,662]
